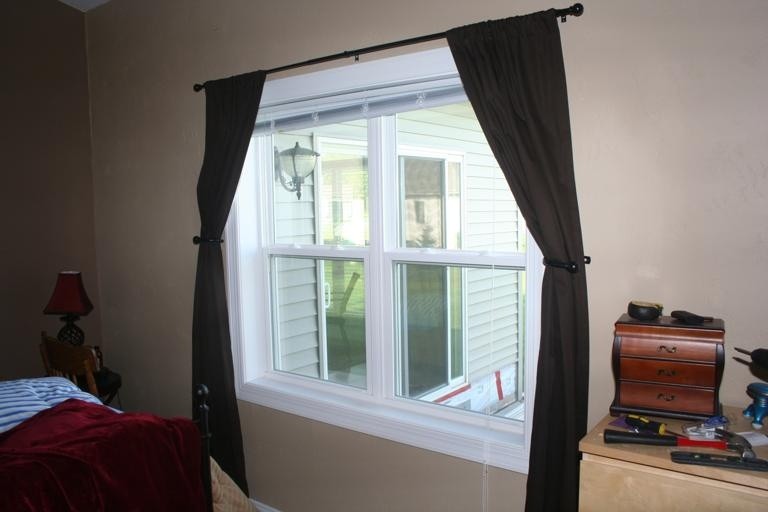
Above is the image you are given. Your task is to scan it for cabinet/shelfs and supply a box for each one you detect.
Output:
[576,404,767,511]
[609,313,726,423]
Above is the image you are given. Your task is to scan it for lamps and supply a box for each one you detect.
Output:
[44,269,94,347]
[274,141,320,200]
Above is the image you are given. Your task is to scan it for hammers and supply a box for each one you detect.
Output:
[604,427,756,459]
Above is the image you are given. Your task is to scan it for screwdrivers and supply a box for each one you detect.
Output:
[625,414,689,438]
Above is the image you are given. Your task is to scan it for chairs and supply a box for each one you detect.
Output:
[39,343,121,409]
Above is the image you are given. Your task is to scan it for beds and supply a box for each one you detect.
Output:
[0,375,217,510]
